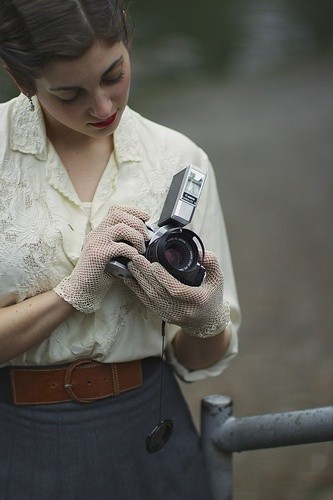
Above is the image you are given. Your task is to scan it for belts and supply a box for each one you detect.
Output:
[10,358,144,405]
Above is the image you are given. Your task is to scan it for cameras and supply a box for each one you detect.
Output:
[106,164,207,286]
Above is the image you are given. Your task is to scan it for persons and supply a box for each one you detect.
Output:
[0,0,239,499]
